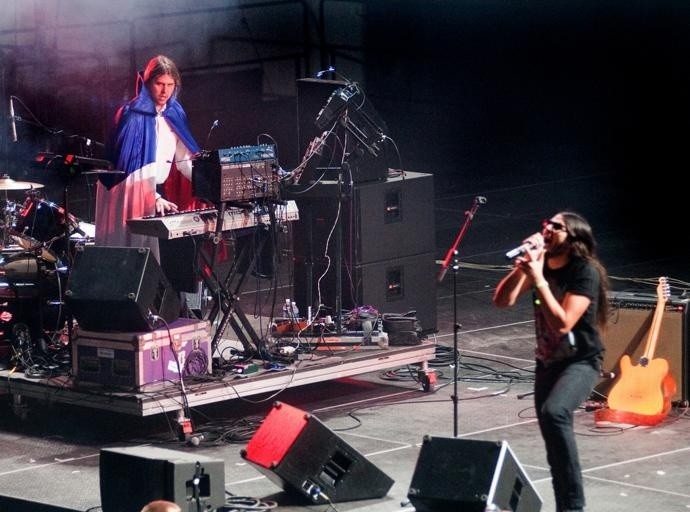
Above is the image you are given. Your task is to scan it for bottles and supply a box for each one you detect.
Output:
[283,298,299,323]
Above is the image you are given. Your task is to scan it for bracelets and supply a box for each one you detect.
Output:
[534,280,548,290]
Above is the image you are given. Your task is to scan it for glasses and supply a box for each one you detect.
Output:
[543,219,566,230]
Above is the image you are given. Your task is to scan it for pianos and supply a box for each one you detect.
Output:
[125,200,300,242]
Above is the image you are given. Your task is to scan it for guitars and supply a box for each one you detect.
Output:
[605,276,672,416]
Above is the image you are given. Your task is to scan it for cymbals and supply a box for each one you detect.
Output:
[83,169,125,176]
[0,178,45,189]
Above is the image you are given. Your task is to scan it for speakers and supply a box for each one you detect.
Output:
[64,246,180,332]
[595,291,690,404]
[294,170,440,334]
[240,401,395,504]
[407,435,544,512]
[99,445,224,512]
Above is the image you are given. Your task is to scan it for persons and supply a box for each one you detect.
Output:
[493,212,611,511]
[96,54,229,315]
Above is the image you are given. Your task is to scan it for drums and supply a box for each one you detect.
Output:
[0,233,44,274]
[65,232,96,256]
[0,283,45,342]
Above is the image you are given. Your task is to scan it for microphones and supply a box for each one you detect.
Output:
[10,99,18,143]
[506,236,543,259]
[600,372,615,378]
